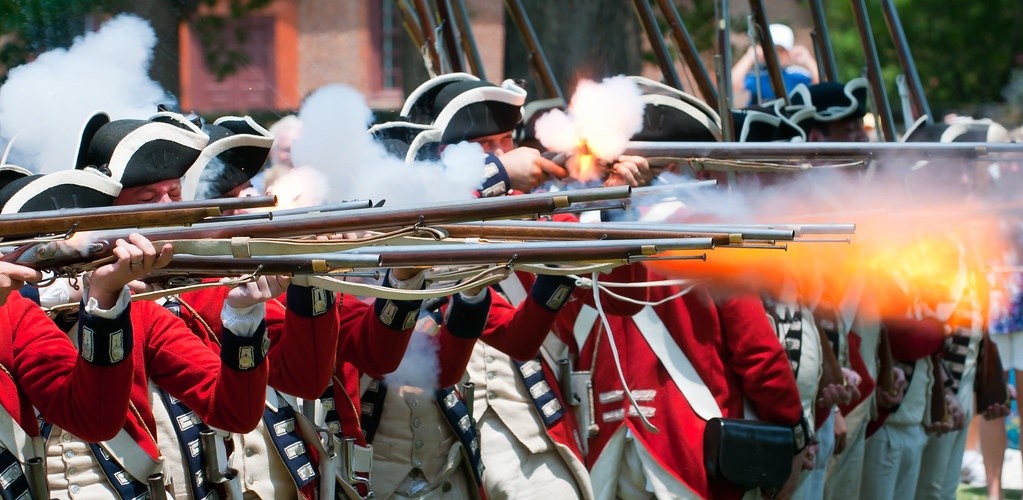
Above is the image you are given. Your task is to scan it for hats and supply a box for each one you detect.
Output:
[402,72,527,143]
[730,98,816,145]
[899,115,990,170]
[788,77,868,124]
[0,165,124,241]
[77,112,210,190]
[516,97,565,153]
[366,122,440,176]
[176,115,274,203]
[622,74,722,143]
[767,23,794,54]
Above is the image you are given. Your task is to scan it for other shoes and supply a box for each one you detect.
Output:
[959,447,983,482]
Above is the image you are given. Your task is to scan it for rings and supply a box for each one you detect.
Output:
[633,169,639,175]
[131,261,143,266]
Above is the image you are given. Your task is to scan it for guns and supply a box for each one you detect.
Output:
[0,141,1023,288]
[396,0,934,141]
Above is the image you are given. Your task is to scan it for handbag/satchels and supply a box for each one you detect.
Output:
[703,416,793,500]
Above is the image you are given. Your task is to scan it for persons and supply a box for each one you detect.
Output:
[0,72,1023,500]
[730,23,818,109]
